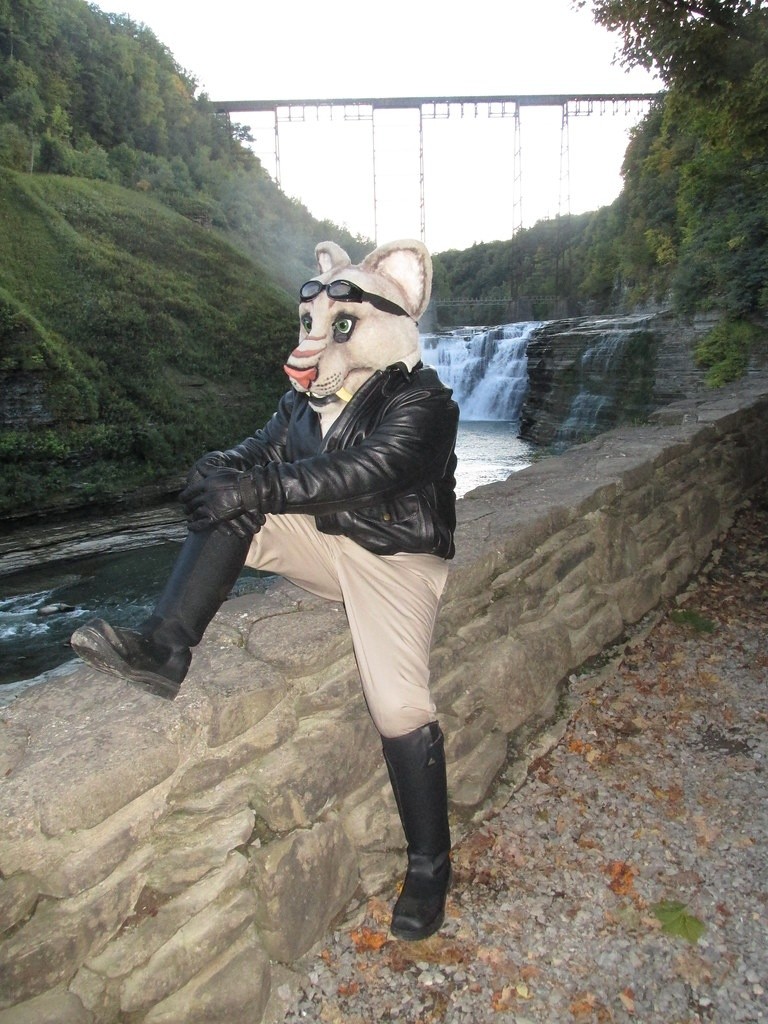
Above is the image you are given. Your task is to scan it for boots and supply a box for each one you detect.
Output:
[71,515,254,702]
[380,720,451,941]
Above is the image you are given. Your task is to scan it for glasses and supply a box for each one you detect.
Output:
[300,279,363,303]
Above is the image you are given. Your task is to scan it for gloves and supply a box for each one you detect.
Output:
[177,461,285,533]
[187,449,266,537]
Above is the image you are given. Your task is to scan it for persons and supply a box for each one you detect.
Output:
[71,240,451,941]
